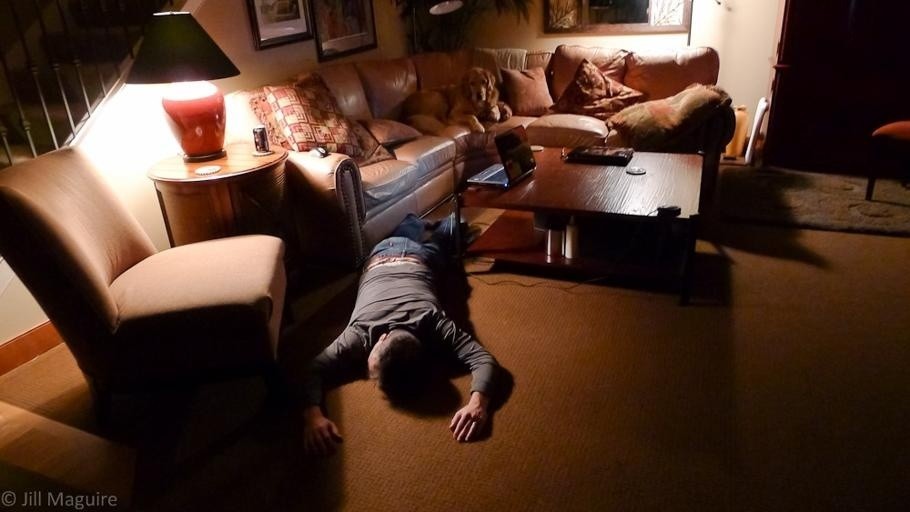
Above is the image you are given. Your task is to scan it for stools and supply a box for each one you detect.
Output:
[147,147,296,249]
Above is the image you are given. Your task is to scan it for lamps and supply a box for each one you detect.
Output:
[410,0,464,52]
[124,12,241,163]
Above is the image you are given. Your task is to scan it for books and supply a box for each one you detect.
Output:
[568,145,633,165]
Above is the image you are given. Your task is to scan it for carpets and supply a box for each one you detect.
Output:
[736,167,909,232]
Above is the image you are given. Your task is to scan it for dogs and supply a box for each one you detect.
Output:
[401,67,502,134]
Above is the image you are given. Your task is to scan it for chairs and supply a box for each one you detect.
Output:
[864,121,909,200]
[0,146,288,432]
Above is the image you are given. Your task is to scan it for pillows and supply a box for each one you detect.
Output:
[499,63,554,115]
[611,82,728,146]
[356,117,421,145]
[255,79,365,159]
[549,59,643,120]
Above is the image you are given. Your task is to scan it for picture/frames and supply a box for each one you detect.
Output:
[308,0,377,62]
[245,2,314,51]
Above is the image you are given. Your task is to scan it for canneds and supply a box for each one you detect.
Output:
[253,126,269,153]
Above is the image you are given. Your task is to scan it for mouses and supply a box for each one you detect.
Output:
[309,146,329,158]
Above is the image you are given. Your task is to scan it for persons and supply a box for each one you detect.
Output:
[301,212,498,458]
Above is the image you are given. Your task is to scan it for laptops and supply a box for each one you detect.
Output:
[466,124,537,189]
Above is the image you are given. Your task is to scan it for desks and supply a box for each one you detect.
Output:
[460,149,707,277]
